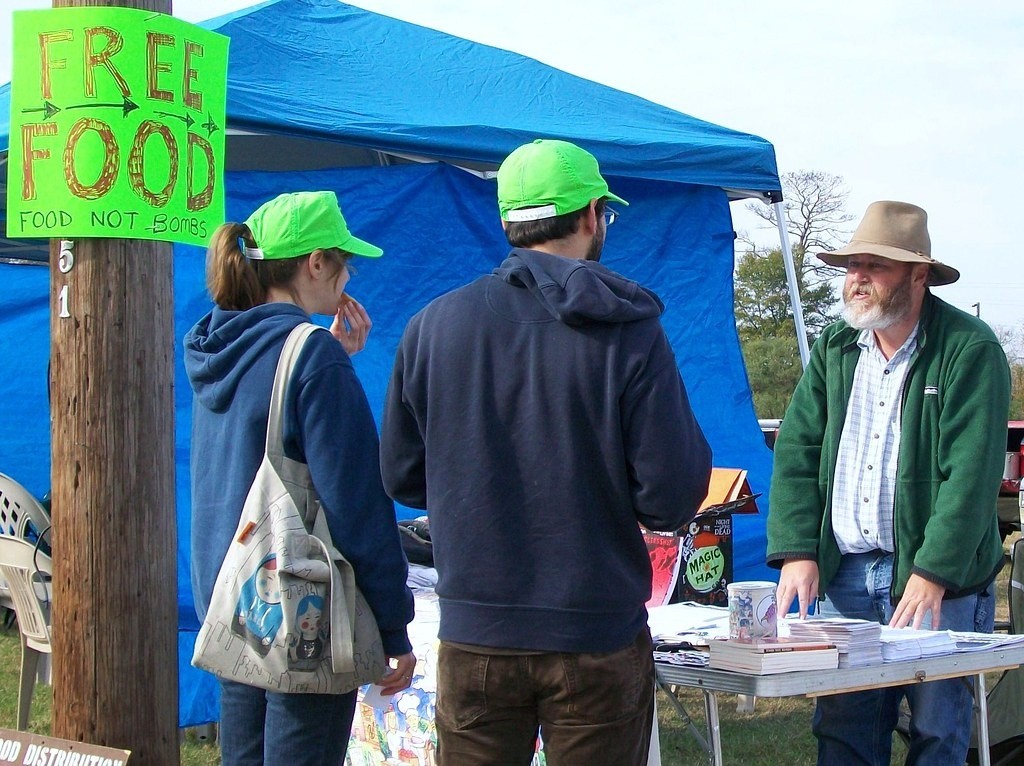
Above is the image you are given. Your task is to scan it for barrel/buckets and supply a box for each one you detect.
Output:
[1003,452,1018,479]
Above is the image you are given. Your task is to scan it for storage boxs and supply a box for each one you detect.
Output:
[638,467,763,608]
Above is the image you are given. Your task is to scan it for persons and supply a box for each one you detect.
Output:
[766,201,1013,766]
[382,141,714,766]
[183,192,416,766]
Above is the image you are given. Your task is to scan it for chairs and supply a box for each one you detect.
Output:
[0,472,55,734]
[896,538,1024,765]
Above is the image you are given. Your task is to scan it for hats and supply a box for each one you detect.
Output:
[818,201,960,288]
[497,139,630,221]
[237,190,383,261]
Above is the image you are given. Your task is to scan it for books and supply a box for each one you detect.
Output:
[647,602,1024,676]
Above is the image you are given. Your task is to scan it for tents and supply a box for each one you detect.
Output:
[1,0,816,724]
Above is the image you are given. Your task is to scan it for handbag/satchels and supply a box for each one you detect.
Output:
[190,320,391,696]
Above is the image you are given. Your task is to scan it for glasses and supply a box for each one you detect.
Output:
[601,202,620,226]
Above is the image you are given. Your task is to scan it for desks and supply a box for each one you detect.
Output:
[339,601,1024,766]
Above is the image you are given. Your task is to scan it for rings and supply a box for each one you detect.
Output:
[403,675,410,681]
